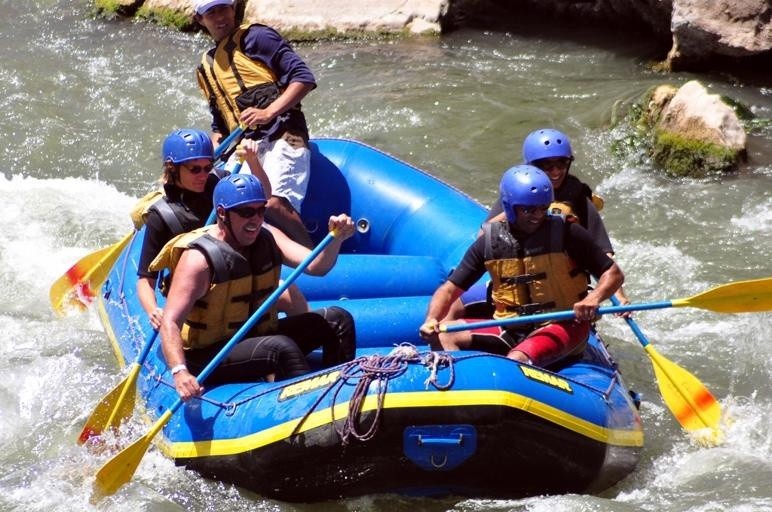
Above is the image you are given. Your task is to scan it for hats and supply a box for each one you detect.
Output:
[193,0,236,15]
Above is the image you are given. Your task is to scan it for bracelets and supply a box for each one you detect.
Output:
[171,363,187,375]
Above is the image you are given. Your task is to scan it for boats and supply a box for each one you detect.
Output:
[93,139,644,502]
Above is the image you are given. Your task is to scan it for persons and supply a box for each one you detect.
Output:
[478,128,634,321]
[418,165,625,375]
[159,175,359,404]
[137,128,313,335]
[191,1,316,251]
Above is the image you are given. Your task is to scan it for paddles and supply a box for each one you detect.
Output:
[50,124,257,317]
[79,142,246,446]
[432,278,771,332]
[90,229,338,504]
[597,274,733,447]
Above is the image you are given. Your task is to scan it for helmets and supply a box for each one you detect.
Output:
[162,127,216,166]
[499,163,556,223]
[521,127,574,166]
[212,173,271,213]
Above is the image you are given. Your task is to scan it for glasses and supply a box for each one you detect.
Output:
[181,162,214,175]
[519,204,549,214]
[230,205,267,219]
